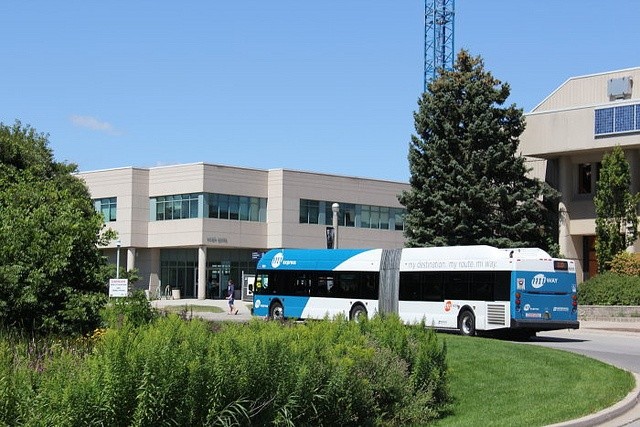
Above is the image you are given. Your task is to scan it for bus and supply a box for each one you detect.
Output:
[250,243,579,337]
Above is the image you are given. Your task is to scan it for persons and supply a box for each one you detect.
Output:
[328,288,335,297]
[226,279,239,316]
[206,278,214,299]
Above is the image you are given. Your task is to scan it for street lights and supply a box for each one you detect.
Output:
[116,238,121,279]
[331,202,340,249]
